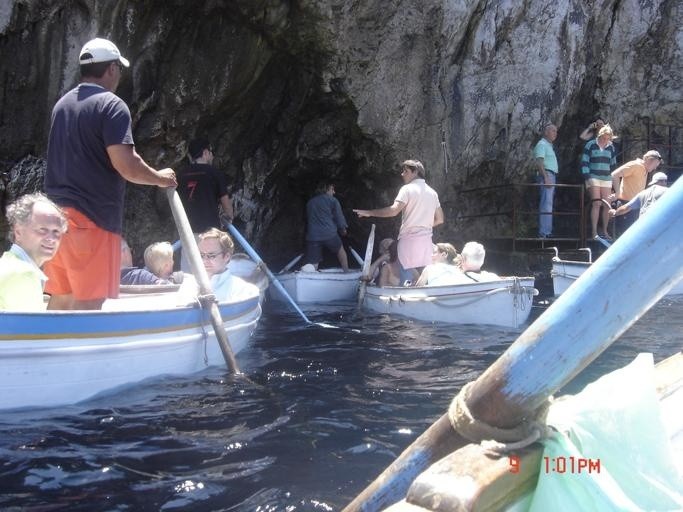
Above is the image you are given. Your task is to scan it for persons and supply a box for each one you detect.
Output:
[361,233,502,287]
[180,227,246,304]
[43,37,178,310]
[353,159,444,286]
[304,181,349,272]
[119,242,184,284]
[119,239,133,267]
[579,113,667,240]
[0,190,69,313]
[532,125,560,246]
[180,136,234,273]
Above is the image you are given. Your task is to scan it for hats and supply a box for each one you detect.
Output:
[78,38,130,68]
[648,172,667,186]
[644,150,664,165]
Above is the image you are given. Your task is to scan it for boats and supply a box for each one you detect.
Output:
[363,276,539,327]
[550,257,683,300]
[0,286,261,410]
[267,267,361,302]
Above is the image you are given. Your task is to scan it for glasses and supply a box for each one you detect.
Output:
[200,250,224,260]
[651,155,663,160]
[112,60,124,72]
